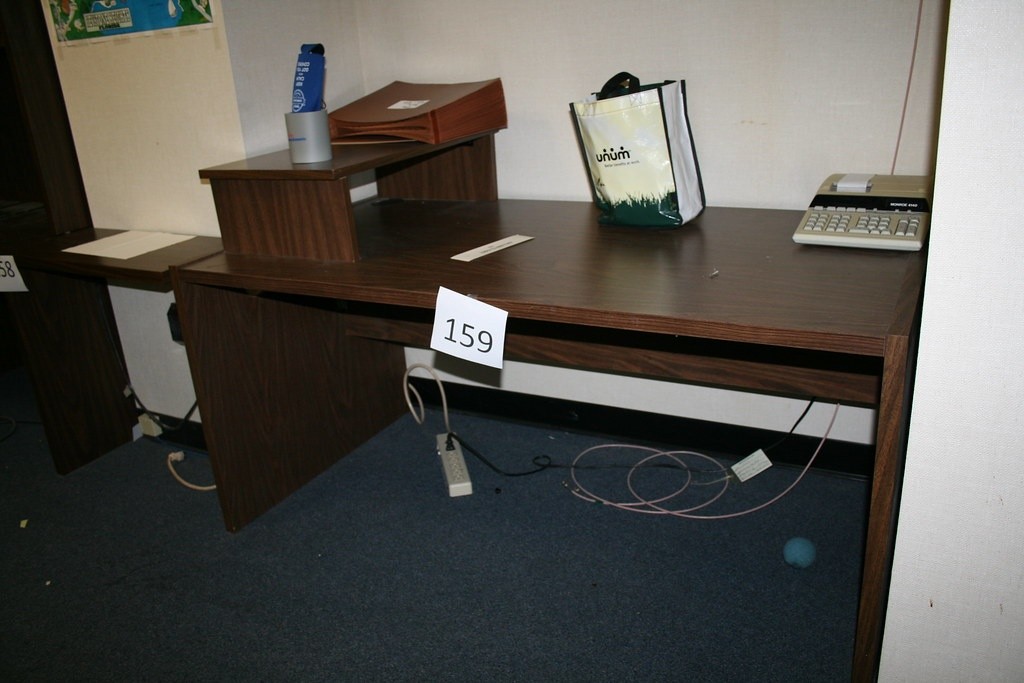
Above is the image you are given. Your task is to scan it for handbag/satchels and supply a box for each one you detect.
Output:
[568,71,706,228]
[330,78,508,147]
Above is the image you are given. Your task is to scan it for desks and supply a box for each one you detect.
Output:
[0,226,226,477]
[172,136,930,683]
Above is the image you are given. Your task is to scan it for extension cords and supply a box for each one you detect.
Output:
[436,433,473,497]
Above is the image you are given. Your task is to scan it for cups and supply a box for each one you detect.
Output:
[284,109,334,164]
